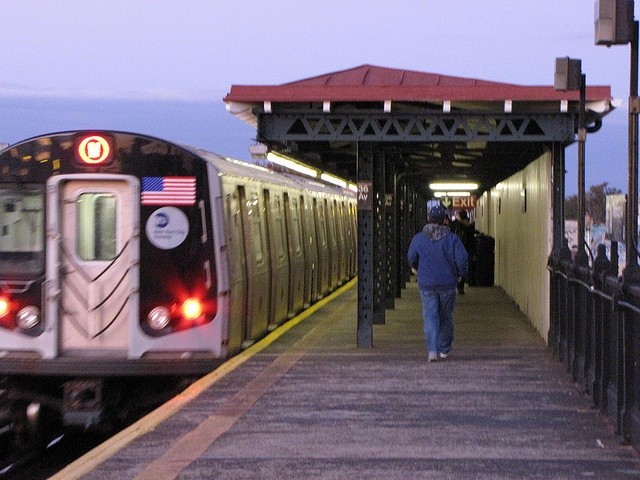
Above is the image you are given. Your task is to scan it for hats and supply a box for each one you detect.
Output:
[459,210,469,220]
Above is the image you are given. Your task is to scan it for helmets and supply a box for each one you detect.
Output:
[430,204,448,223]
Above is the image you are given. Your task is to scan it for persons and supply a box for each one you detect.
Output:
[592,244,610,292]
[407,205,469,362]
[451,211,467,293]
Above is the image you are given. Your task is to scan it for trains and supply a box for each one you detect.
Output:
[0,128,356,444]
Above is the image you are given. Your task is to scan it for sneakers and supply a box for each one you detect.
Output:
[428,350,438,361]
[440,349,453,358]
[457,287,465,294]
